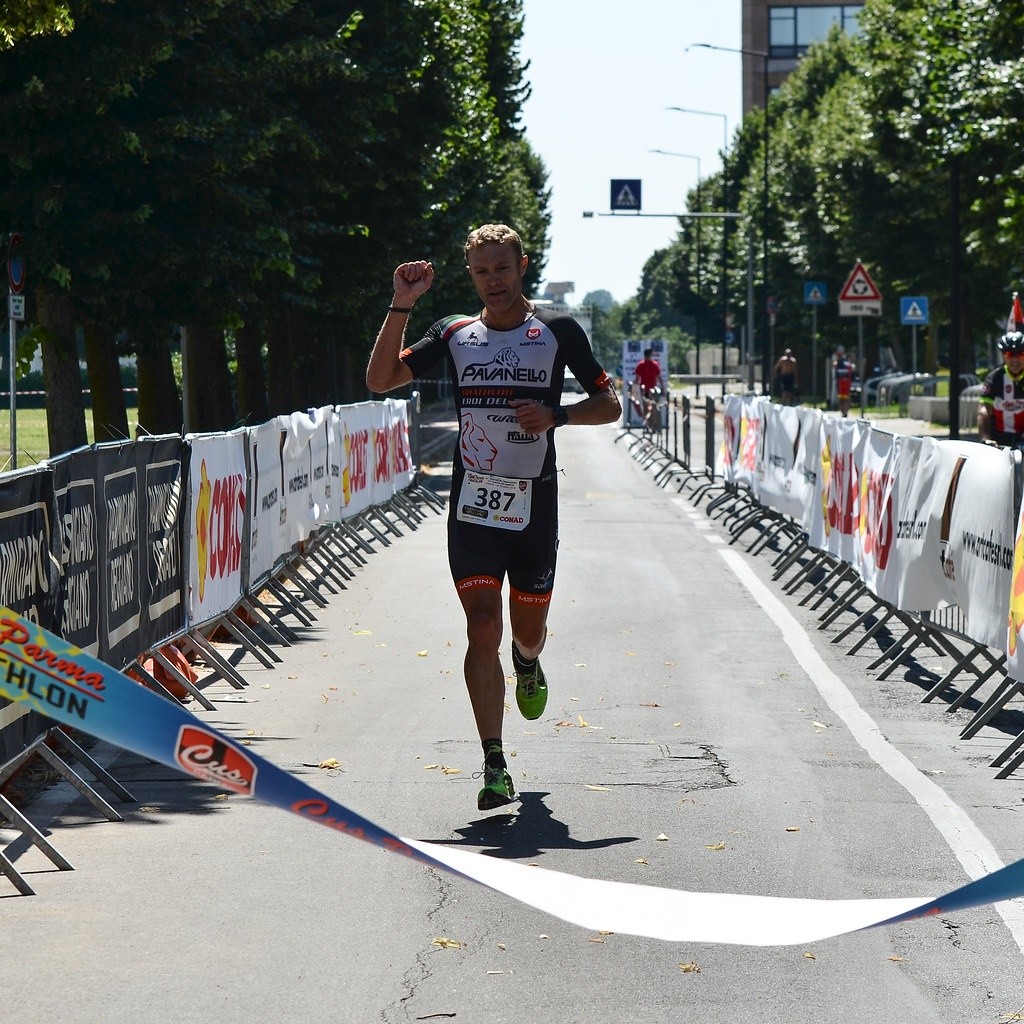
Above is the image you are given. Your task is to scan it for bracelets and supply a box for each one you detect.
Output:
[388,306,412,313]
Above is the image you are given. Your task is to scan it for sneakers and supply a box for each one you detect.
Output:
[474,764,520,811]
[513,641,548,720]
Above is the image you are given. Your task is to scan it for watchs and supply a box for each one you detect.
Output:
[551,405,568,428]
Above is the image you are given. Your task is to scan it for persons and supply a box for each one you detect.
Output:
[366,224,621,810]
[834,355,853,418]
[775,349,799,407]
[977,332,1024,544]
[635,349,665,432]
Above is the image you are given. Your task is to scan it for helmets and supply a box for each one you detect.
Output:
[997,331,1024,352]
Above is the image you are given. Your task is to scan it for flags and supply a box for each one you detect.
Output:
[1006,297,1024,332]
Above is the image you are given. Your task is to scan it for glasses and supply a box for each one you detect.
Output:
[1002,351,1024,360]
